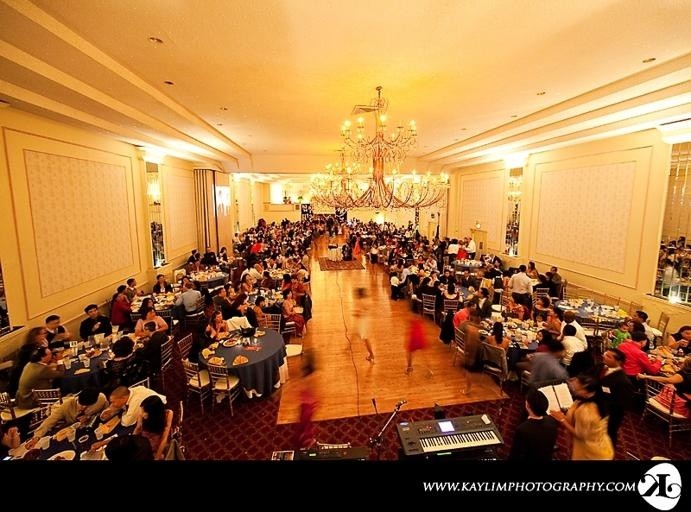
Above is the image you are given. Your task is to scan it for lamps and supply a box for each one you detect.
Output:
[280,86,452,210]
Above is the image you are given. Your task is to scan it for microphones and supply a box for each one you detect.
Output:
[396,399,407,407]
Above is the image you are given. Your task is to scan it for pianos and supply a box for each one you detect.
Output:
[396,413,505,457]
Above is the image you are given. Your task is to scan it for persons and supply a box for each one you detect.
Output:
[474,287,492,318]
[509,390,559,460]
[489,252,503,266]
[527,339,568,392]
[117,285,133,302]
[127,278,144,298]
[258,258,267,270]
[15,347,65,409]
[617,331,663,391]
[440,269,457,284]
[254,296,265,327]
[134,321,170,369]
[533,311,545,327]
[231,281,242,296]
[545,272,558,297]
[533,275,548,293]
[416,255,425,264]
[638,358,691,417]
[106,336,153,391]
[218,287,232,305]
[175,282,204,318]
[403,298,430,376]
[100,386,168,427]
[45,315,70,347]
[80,304,112,343]
[543,308,561,339]
[291,258,307,271]
[632,310,655,346]
[425,254,437,269]
[512,304,532,321]
[180,275,190,282]
[526,261,539,279]
[427,271,440,287]
[282,274,292,291]
[211,311,228,332]
[653,236,691,297]
[188,320,218,363]
[491,257,504,304]
[428,281,442,310]
[557,311,588,349]
[0,420,22,460]
[261,271,275,288]
[282,289,306,336]
[442,284,459,313]
[560,325,585,365]
[153,274,173,292]
[132,395,174,460]
[458,311,483,394]
[135,307,168,337]
[272,254,284,269]
[187,246,228,273]
[630,321,650,351]
[232,293,249,313]
[232,214,477,260]
[462,270,474,287]
[192,281,202,293]
[17,326,49,367]
[397,258,406,298]
[531,296,552,322]
[516,329,553,378]
[105,435,154,461]
[668,326,691,355]
[486,321,508,368]
[300,346,317,450]
[549,376,615,460]
[609,322,632,349]
[418,277,431,299]
[551,266,561,298]
[601,348,634,450]
[389,266,399,300]
[453,301,477,331]
[110,293,134,331]
[508,264,533,307]
[224,283,237,300]
[345,288,374,364]
[24,387,109,450]
[242,274,253,293]
[255,261,264,273]
[137,298,158,317]
[240,260,263,285]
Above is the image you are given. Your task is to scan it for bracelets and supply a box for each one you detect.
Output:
[560,418,566,423]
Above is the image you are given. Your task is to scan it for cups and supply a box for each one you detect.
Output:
[66,429,75,442]
[40,437,50,450]
[63,360,72,370]
[94,427,103,440]
[83,358,91,367]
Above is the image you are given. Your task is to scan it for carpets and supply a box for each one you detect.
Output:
[318,257,366,271]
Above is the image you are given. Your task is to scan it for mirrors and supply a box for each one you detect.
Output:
[1,265,10,336]
[651,141,691,306]
[146,162,170,269]
[503,176,520,257]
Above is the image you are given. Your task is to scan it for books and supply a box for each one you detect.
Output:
[537,383,574,416]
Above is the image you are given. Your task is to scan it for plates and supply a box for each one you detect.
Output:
[223,339,238,347]
[78,434,89,443]
[80,449,106,461]
[47,449,76,461]
